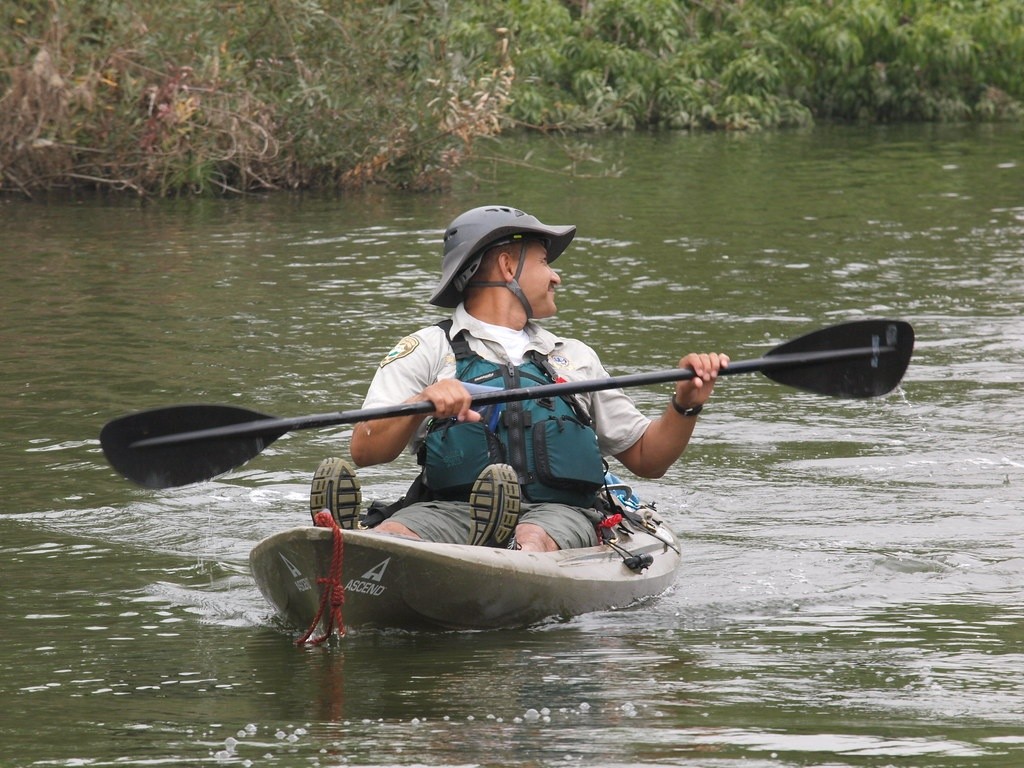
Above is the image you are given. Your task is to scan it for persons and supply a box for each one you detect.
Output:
[300,202,730,590]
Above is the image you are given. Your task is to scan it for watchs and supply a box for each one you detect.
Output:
[666,391,705,418]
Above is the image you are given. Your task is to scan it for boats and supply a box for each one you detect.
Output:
[250,500,683,632]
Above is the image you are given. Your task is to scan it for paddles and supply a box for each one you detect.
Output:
[99,317,916,491]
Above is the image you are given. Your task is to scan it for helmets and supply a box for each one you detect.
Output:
[427,205,577,308]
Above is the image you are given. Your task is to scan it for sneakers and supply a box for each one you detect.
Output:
[465,463,522,550]
[309,457,361,530]
